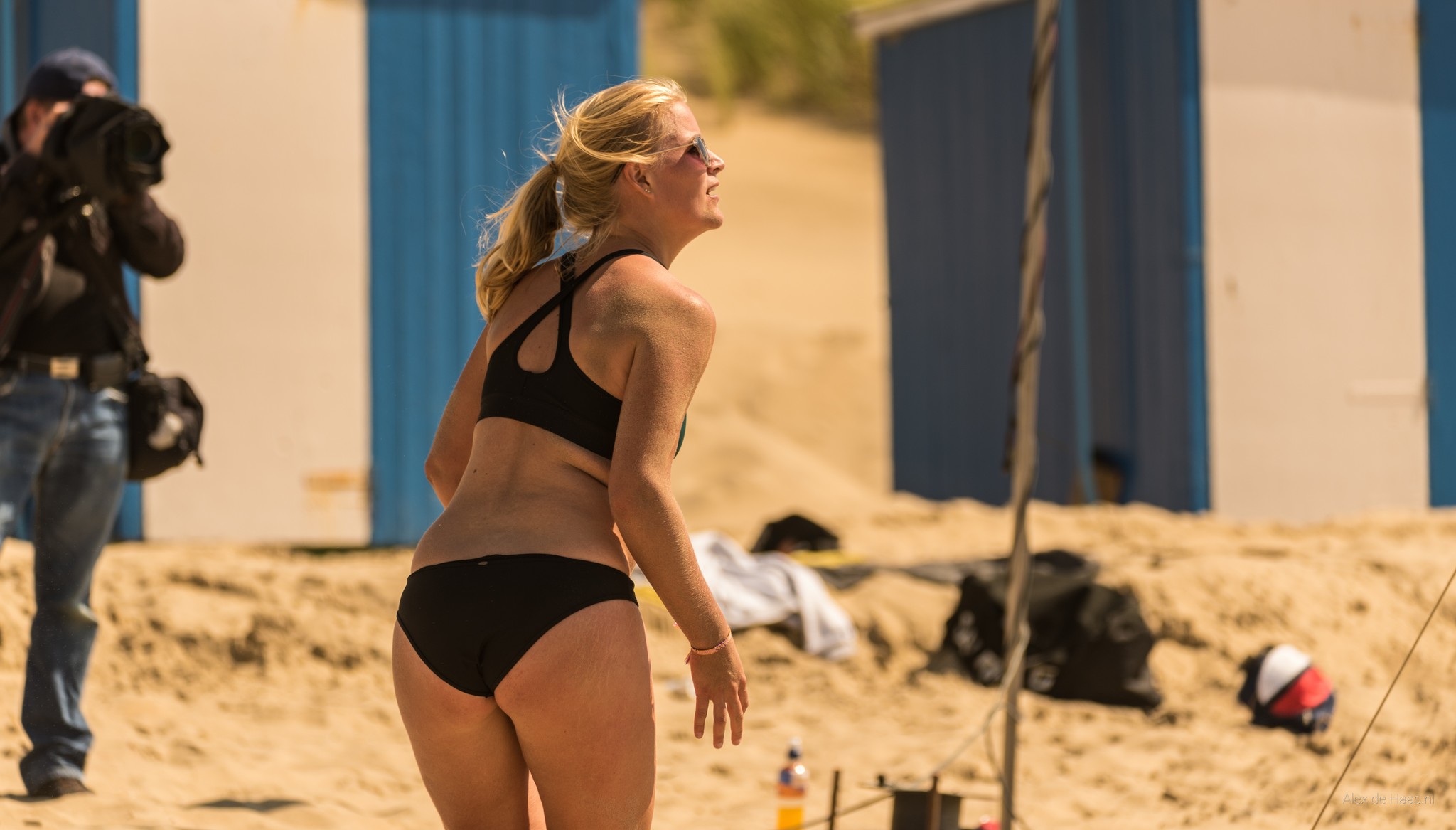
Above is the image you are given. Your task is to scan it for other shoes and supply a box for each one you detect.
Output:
[31,779,91,797]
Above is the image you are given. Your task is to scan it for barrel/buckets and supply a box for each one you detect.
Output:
[891,790,962,830]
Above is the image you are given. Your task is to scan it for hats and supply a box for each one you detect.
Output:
[9,49,115,118]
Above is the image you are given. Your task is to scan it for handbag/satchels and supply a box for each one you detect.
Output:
[131,367,208,480]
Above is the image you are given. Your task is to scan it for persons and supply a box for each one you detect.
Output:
[0,50,184,800]
[389,75,750,830]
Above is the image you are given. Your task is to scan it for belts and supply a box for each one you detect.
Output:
[25,354,89,381]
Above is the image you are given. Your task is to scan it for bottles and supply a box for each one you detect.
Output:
[775,748,808,830]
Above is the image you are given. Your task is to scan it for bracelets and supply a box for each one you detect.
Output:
[685,625,732,664]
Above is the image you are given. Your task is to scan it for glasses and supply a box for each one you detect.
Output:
[609,135,712,186]
